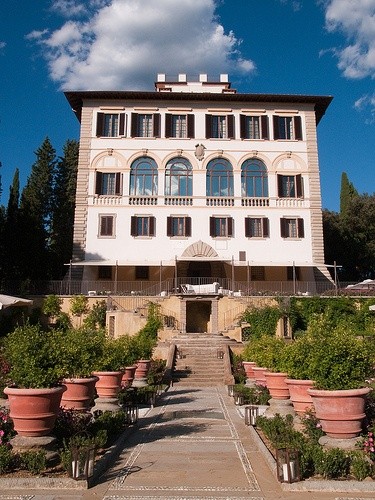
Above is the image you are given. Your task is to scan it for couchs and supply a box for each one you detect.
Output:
[186,283,219,293]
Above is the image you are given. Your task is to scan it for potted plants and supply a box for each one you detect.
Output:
[283,336,316,415]
[3,318,68,436]
[93,336,121,400]
[134,331,151,378]
[52,330,105,414]
[253,342,267,387]
[114,336,138,391]
[265,340,291,399]
[244,342,257,378]
[306,334,371,440]
